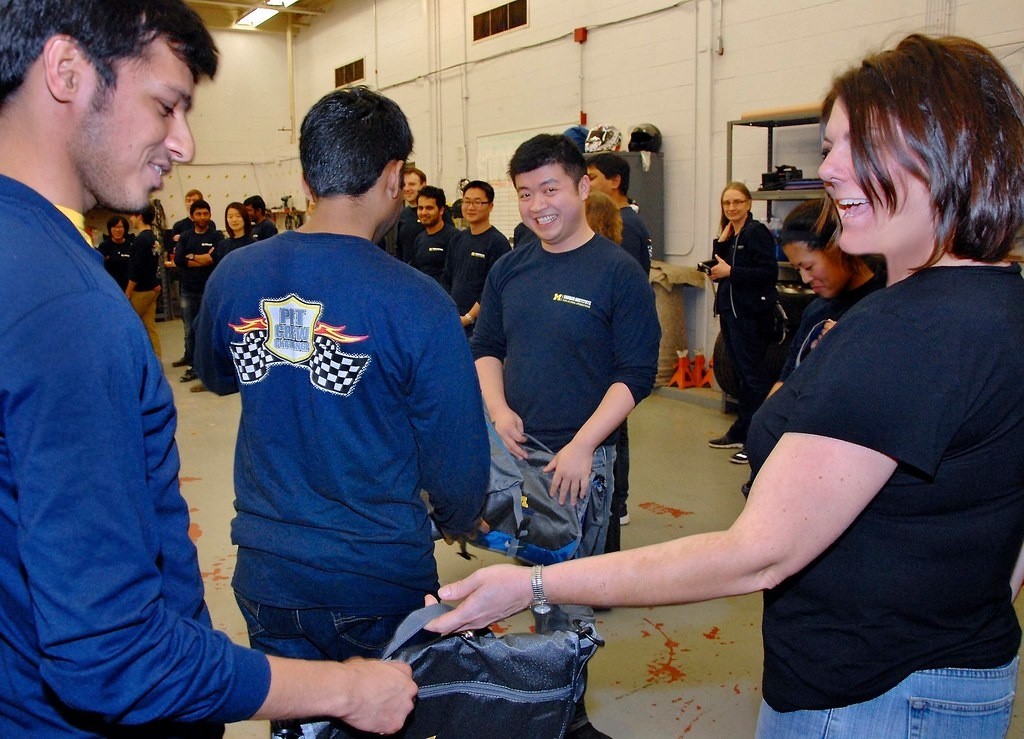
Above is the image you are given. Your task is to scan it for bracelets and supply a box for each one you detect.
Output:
[465,314,475,324]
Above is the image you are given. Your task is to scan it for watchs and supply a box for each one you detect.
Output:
[529,564,554,616]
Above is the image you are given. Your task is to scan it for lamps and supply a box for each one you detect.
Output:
[235,0,298,26]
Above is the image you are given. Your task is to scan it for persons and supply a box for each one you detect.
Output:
[412,186,461,282]
[513,222,539,249]
[0,0,417,739]
[178,202,259,392]
[243,195,278,243]
[124,203,162,361]
[421,35,1023,739]
[437,180,513,342]
[584,152,653,558]
[170,189,215,254]
[97,215,134,296]
[189,82,494,738]
[395,167,455,267]
[470,135,661,617]
[171,200,221,367]
[701,179,887,464]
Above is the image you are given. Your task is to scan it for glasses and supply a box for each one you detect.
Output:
[722,199,748,206]
[461,198,491,208]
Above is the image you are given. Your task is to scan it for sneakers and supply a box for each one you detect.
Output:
[729,445,749,464]
[708,434,744,449]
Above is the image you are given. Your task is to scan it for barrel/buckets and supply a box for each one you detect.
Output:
[652,281,685,380]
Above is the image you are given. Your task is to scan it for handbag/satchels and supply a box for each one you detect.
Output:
[762,165,802,190]
[464,433,597,565]
[770,299,789,345]
[298,603,605,739]
[419,392,523,541]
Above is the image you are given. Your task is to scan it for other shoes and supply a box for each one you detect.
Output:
[172,356,188,366]
[619,504,631,525]
[191,384,208,392]
[180,368,198,382]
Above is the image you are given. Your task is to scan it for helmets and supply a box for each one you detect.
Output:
[562,127,589,152]
[628,124,662,152]
[585,124,622,153]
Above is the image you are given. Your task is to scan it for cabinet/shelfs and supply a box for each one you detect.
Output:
[723,105,856,268]
